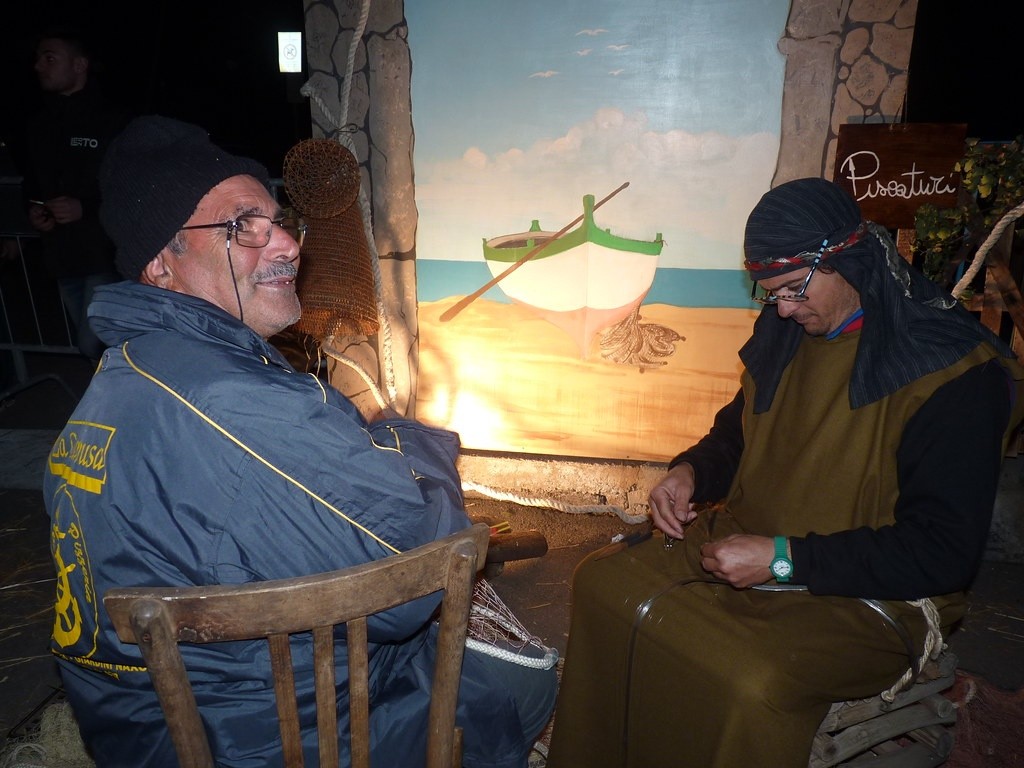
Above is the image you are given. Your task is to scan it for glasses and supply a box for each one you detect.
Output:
[749,226,849,309]
[178,208,307,248]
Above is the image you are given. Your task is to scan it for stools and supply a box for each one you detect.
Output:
[806,648,959,768]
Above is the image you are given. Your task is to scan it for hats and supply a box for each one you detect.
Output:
[99,117,271,281]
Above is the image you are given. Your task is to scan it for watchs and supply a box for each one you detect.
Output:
[770,535,793,583]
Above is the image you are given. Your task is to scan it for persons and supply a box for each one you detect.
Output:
[20,26,142,370]
[546,177,1024,768]
[41,116,559,768]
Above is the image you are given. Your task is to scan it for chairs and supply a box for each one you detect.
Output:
[101,522,490,768]
